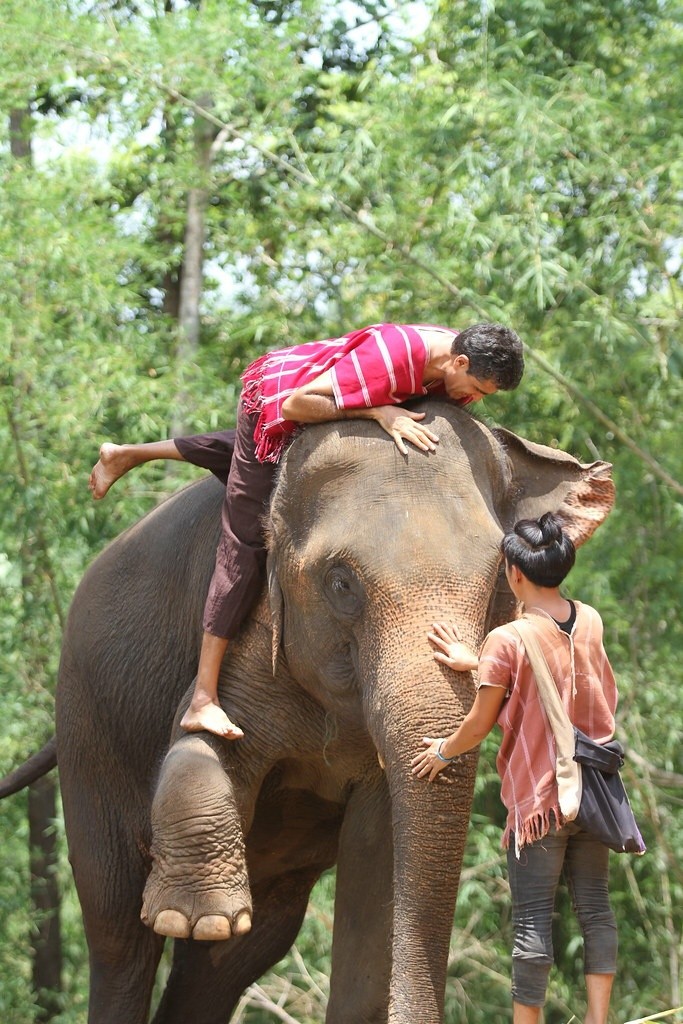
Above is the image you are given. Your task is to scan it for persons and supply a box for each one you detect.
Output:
[410,511,618,1024]
[88,323,525,740]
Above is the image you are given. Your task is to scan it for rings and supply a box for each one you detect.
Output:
[458,639,463,641]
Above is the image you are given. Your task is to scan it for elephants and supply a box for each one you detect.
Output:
[0,401,614,1024]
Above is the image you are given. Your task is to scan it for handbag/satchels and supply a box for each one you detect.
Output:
[552,723,647,857]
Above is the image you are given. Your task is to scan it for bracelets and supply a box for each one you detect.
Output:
[438,741,456,762]
